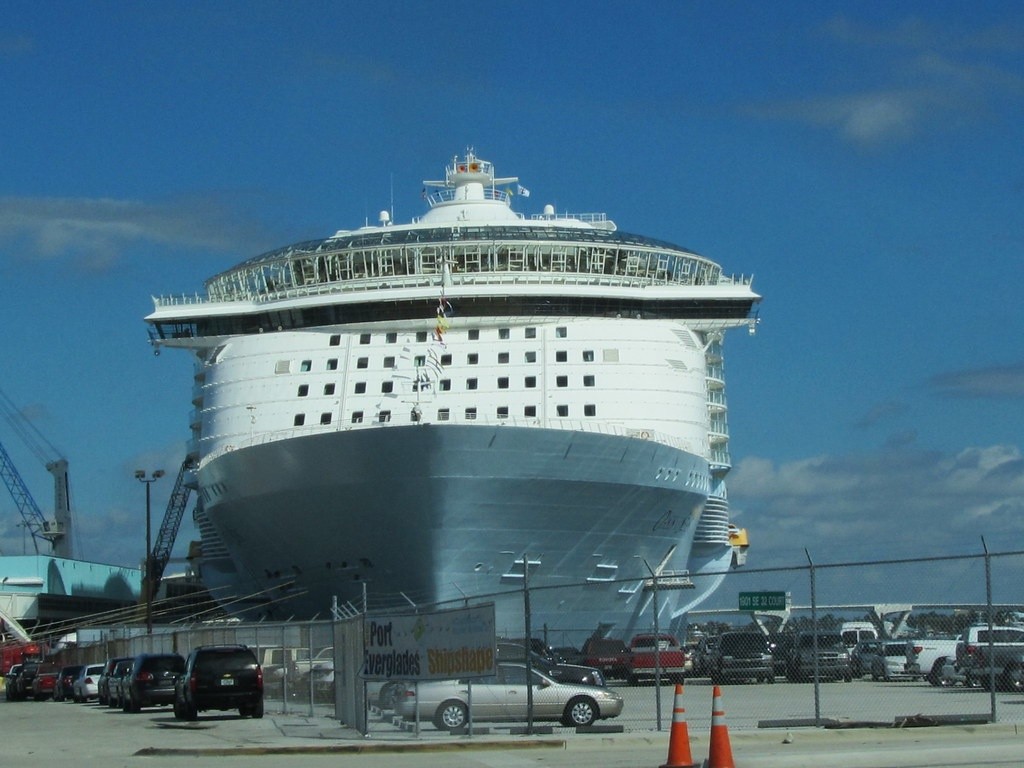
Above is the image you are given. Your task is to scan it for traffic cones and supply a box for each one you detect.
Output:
[660,683,694,768]
[702,685,734,768]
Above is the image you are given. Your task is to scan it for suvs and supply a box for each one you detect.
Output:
[174,644,264,719]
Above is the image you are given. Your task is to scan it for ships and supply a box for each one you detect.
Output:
[142,148,763,642]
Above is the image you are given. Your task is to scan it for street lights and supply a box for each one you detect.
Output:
[136,470,165,651]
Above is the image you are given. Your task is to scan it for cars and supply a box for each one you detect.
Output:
[265,607,1024,728]
[5,652,185,714]
